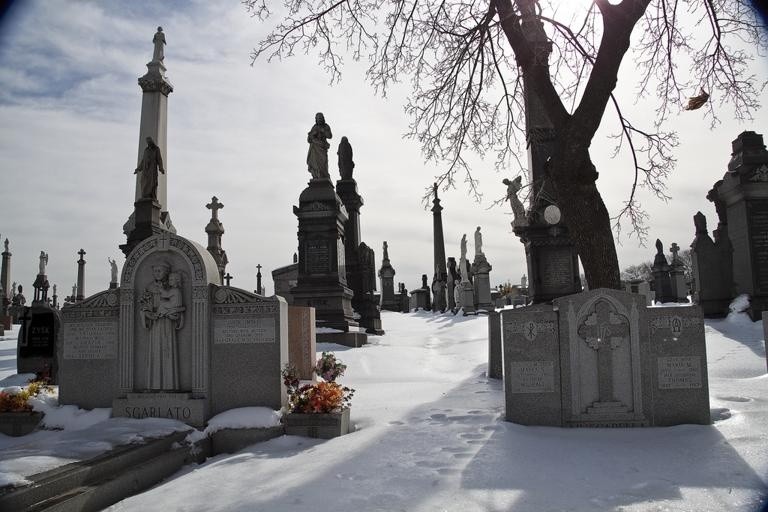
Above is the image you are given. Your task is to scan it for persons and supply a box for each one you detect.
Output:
[474,226,483,256]
[152,26,167,62]
[140,260,185,393]
[108,256,118,283]
[306,112,333,179]
[459,233,468,259]
[39,250,48,275]
[133,135,165,200]
[521,274,527,288]
[502,175,526,220]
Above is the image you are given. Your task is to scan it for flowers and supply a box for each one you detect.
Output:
[279,350,356,415]
[0,372,57,413]
